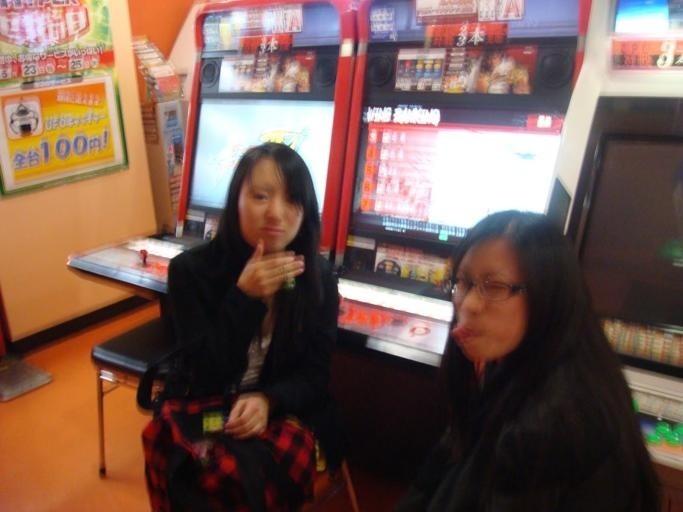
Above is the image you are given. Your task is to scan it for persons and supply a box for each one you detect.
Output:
[457,44,532,95]
[419,209,664,510]
[244,52,311,93]
[140,143,362,511]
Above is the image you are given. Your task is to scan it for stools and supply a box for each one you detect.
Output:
[91,317,168,477]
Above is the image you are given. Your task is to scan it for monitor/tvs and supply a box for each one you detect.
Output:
[343,104,580,248]
[615,0,669,36]
[187,93,338,224]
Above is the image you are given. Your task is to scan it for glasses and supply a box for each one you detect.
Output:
[436,276,530,302]
